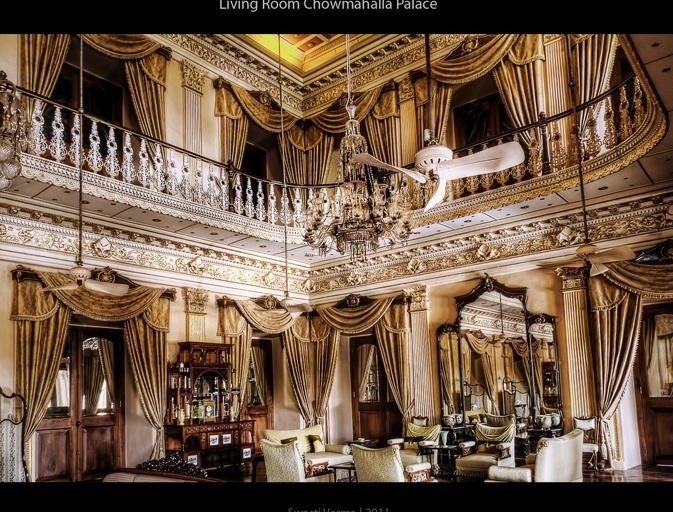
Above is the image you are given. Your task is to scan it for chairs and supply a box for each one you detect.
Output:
[258,413,600,482]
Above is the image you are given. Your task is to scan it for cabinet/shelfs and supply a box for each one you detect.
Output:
[164,341,257,473]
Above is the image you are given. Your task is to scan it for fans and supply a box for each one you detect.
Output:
[38,34,638,319]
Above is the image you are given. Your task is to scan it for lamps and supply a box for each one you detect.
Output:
[0,79,23,192]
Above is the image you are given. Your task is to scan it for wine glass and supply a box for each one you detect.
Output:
[440,431,449,448]
